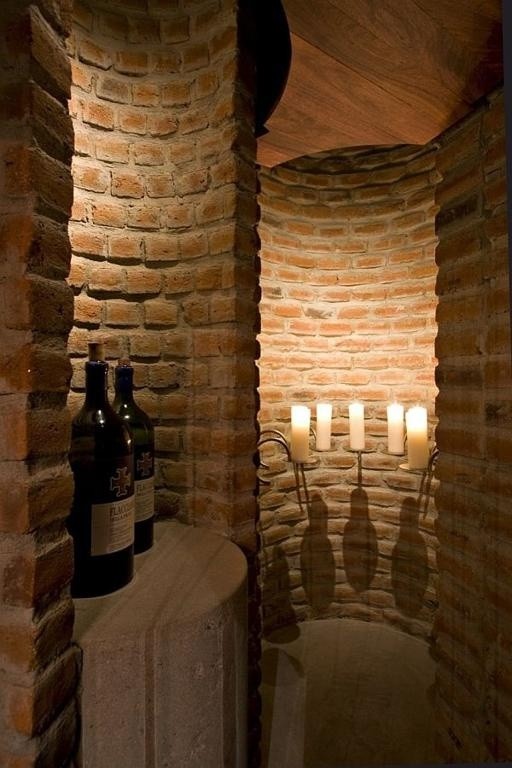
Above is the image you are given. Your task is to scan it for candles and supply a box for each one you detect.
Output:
[349,404,365,450]
[291,405,310,461]
[316,404,332,450]
[387,401,404,453]
[406,406,430,469]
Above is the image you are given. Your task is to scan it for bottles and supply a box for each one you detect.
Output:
[70,339,136,597]
[108,348,156,555]
